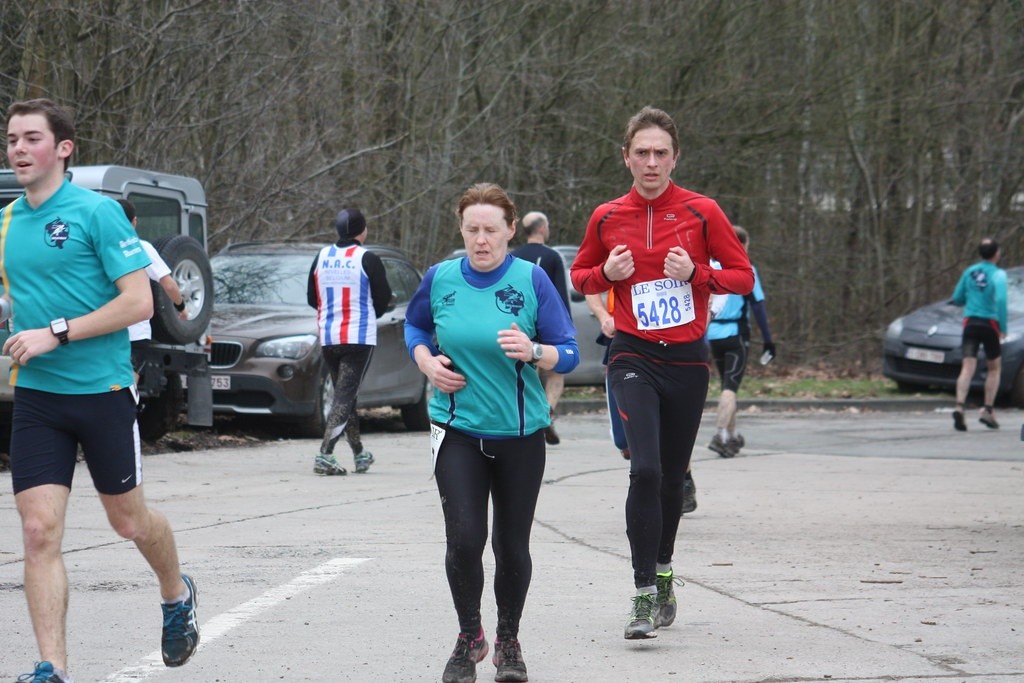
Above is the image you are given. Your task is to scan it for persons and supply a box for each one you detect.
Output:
[952,238,1007,431]
[0,98,201,683]
[115,199,188,386]
[307,208,391,475]
[508,212,573,445]
[402,181,579,683]
[704,226,776,458]
[570,109,756,639]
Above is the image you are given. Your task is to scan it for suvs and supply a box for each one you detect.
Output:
[0,163,215,457]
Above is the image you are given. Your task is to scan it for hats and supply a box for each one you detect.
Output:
[337,207,366,238]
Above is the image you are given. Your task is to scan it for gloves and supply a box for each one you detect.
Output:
[762,341,776,360]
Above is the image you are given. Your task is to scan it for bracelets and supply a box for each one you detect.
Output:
[173,299,185,312]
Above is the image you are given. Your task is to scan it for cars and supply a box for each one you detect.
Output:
[881,266,1024,411]
[444,245,609,386]
[176,239,440,439]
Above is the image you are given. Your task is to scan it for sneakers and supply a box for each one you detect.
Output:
[354,449,374,473]
[656,566,685,626]
[15,661,75,683]
[314,453,347,475]
[442,625,489,683]
[624,593,662,639]
[160,572,200,667]
[492,636,528,682]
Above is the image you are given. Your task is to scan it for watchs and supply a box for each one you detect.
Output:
[48,317,69,346]
[531,341,542,364]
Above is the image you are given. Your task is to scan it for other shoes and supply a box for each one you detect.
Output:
[708,433,744,458]
[620,447,630,460]
[953,411,967,431]
[544,427,559,444]
[682,470,697,513]
[981,408,999,429]
